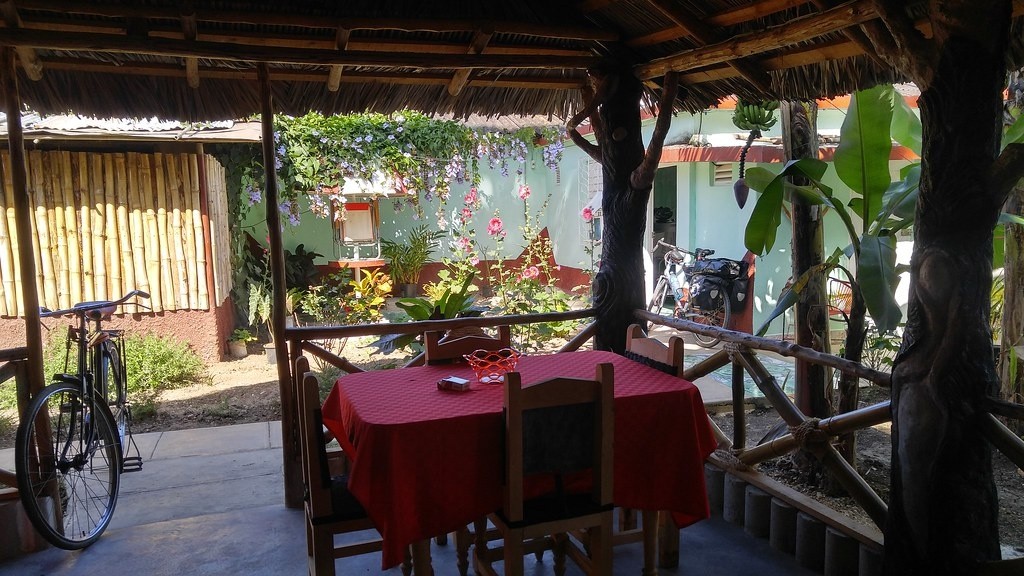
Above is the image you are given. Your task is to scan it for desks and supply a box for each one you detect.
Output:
[319,351,718,576]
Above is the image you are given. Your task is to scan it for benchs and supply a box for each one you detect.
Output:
[783,262,852,339]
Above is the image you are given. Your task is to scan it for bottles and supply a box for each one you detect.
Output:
[670,271,684,300]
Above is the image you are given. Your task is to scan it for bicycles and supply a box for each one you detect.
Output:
[646,238,733,348]
[14,289,153,552]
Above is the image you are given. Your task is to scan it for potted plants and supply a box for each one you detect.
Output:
[285,287,305,327]
[226,329,257,357]
[377,224,449,298]
[249,284,277,364]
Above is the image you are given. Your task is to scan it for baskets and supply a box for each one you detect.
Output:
[463,347,523,384]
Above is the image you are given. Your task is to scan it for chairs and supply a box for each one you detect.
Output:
[473,361,614,575]
[568,324,684,575]
[424,325,544,575]
[295,356,413,576]
